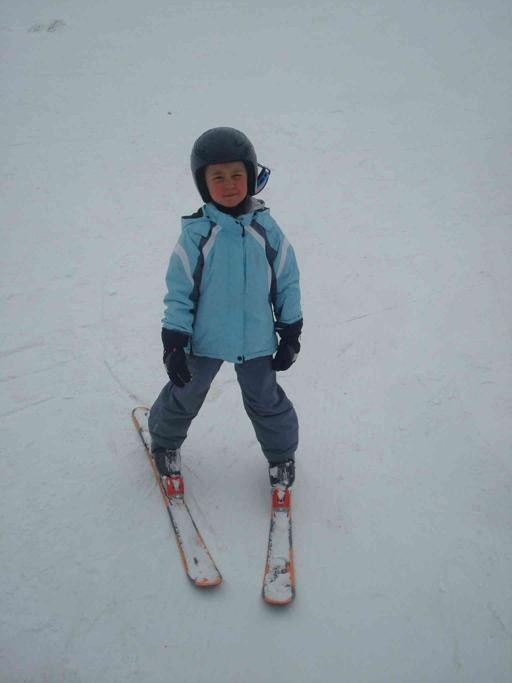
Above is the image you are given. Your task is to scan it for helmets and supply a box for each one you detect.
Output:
[190,127,269,203]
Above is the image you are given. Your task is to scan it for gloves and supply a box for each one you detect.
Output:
[162,346,192,388]
[272,334,301,372]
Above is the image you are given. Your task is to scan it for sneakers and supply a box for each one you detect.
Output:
[154,445,181,476]
[268,457,296,489]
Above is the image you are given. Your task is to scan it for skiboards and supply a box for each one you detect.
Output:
[131,408,295,607]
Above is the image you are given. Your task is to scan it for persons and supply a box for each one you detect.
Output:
[148,127,303,492]
